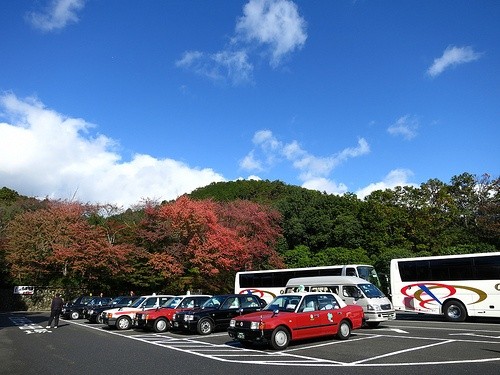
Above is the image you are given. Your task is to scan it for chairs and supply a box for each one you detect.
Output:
[303,300,315,312]
[286,300,298,310]
[187,300,194,308]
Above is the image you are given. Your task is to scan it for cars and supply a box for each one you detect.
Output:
[228,290,364,350]
[61,294,268,335]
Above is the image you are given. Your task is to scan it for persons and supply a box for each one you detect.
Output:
[45,293,63,328]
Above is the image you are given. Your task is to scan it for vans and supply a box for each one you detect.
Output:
[282,276,398,325]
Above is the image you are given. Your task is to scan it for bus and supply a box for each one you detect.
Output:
[389,252,500,320]
[234,264,382,302]
